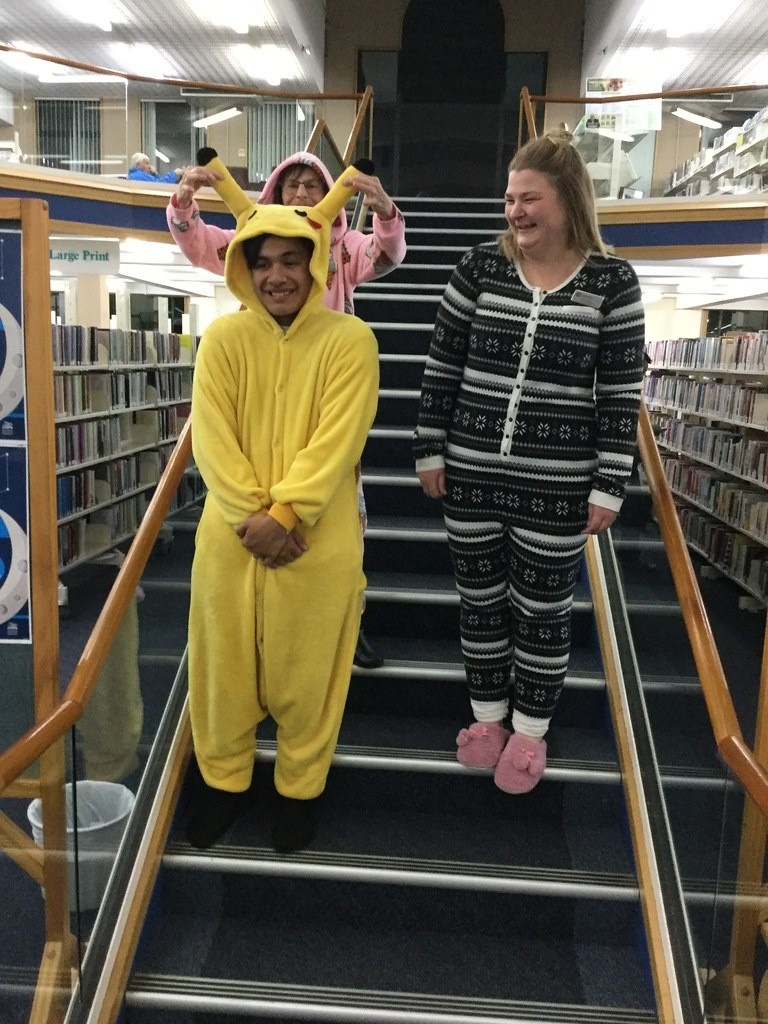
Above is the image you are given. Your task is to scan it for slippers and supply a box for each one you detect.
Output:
[493,733,548,795]
[456,721,511,768]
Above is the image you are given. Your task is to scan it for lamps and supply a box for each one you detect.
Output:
[193,106,244,128]
[670,106,722,130]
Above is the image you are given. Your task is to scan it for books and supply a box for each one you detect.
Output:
[644,329,768,606]
[663,105,768,198]
[50,322,203,572]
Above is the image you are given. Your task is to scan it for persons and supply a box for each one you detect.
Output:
[413,126,653,795]
[164,148,408,849]
[607,78,624,92]
[127,151,186,185]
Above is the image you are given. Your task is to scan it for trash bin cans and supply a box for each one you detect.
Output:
[26,779,137,912]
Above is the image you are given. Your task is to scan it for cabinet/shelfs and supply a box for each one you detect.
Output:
[638,359,768,616]
[52,357,210,579]
[661,108,768,198]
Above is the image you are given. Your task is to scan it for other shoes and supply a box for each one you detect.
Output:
[272,796,317,850]
[185,795,245,850]
